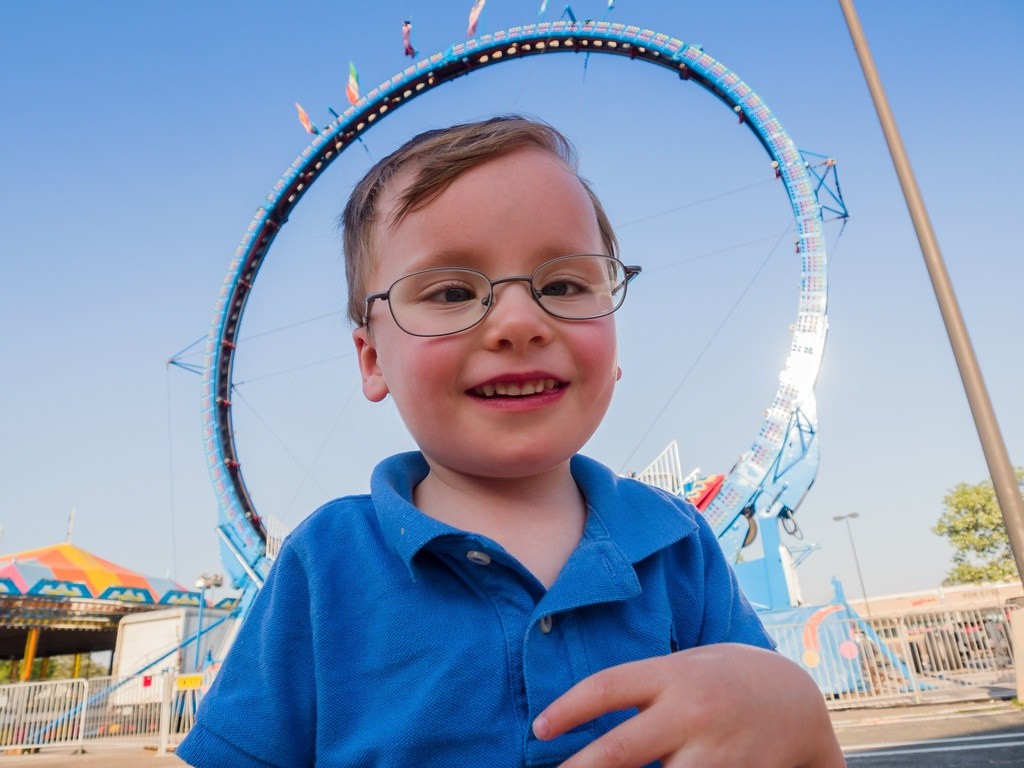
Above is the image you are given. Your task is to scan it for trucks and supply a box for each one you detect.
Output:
[107,605,241,723]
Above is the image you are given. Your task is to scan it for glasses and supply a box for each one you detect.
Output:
[363,254,642,337]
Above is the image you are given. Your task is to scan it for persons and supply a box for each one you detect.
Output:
[177,113,846,768]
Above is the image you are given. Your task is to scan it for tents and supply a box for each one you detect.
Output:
[0,542,240,681]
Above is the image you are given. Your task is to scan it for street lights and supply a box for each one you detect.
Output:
[835,510,874,627]
